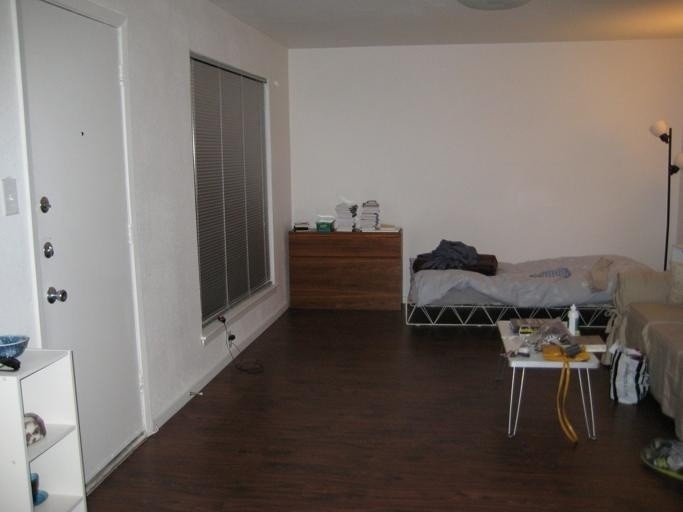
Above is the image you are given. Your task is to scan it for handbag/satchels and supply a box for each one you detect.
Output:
[610,347,649,404]
[543,345,590,361]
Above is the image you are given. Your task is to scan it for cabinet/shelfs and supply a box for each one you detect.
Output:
[1,347,89,512]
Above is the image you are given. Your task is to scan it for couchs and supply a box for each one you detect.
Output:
[604,263,683,444]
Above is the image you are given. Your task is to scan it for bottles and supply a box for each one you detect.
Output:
[567,304,580,337]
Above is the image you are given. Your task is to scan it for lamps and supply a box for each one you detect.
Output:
[649,119,682,270]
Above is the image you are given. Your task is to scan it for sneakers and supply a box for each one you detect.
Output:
[642,438,682,480]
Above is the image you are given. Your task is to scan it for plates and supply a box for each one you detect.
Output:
[34,490,48,507]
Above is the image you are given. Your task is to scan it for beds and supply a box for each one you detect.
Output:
[404,255,650,330]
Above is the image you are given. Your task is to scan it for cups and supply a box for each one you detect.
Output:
[30,472,39,503]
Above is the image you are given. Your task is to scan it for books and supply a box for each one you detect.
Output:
[334,199,380,232]
[293,222,310,233]
[510,318,546,332]
[570,335,606,353]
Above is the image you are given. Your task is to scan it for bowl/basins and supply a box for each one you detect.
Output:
[0,335,30,361]
